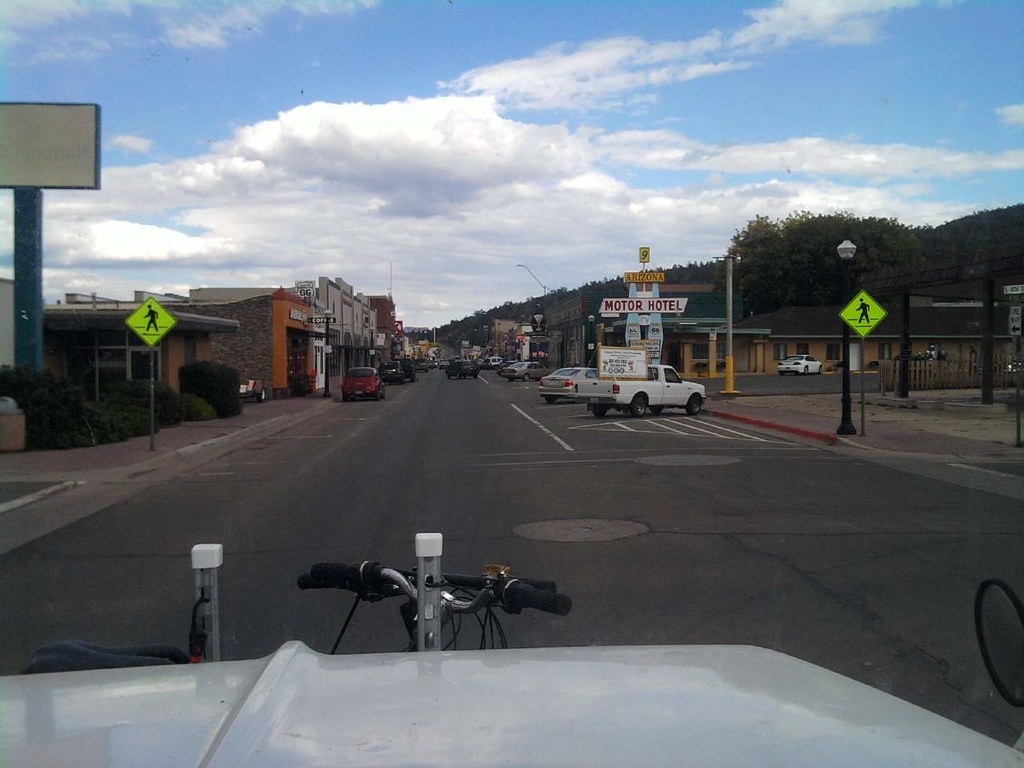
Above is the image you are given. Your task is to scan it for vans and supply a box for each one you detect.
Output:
[482,357,504,370]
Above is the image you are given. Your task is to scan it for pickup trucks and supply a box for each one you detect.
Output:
[567,365,705,418]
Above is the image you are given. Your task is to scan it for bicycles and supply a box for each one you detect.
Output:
[299,562,571,653]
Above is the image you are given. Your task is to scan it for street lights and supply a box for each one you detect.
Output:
[588,315,595,366]
[517,264,547,367]
[837,240,858,435]
[369,327,374,367]
[322,309,333,397]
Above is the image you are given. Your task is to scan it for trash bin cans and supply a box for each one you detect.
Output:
[0,396,26,452]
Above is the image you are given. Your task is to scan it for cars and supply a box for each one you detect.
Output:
[497,360,552,382]
[341,367,385,401]
[234,369,266,402]
[538,367,598,403]
[777,354,823,376]
[379,357,479,385]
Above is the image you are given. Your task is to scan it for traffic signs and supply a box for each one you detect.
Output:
[1009,307,1022,336]
